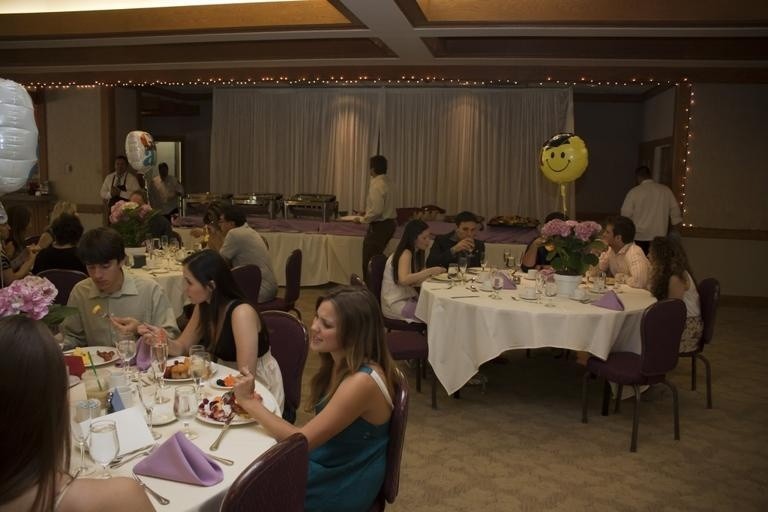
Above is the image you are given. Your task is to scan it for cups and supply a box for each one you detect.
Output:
[479,258,488,272]
[151,337,171,404]
[189,345,205,385]
[504,248,511,264]
[535,270,545,304]
[110,371,132,386]
[116,332,137,387]
[81,370,113,410]
[591,265,625,297]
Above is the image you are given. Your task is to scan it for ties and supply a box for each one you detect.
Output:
[115,175,125,191]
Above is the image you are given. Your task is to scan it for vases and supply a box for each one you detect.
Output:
[124,247,146,257]
[553,275,583,295]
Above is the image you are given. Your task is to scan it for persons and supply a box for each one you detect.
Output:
[590,215,651,289]
[5,205,41,271]
[233,284,409,512]
[203,207,276,303]
[27,200,80,251]
[619,165,684,255]
[0,202,40,285]
[57,226,182,347]
[31,211,89,276]
[425,211,485,267]
[352,156,398,281]
[520,212,571,273]
[203,200,227,251]
[380,219,447,324]
[110,248,285,416]
[647,235,706,354]
[0,314,156,511]
[148,162,185,222]
[99,156,142,225]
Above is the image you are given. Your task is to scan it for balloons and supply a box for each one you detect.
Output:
[1,75,41,199]
[538,132,589,213]
[125,130,157,183]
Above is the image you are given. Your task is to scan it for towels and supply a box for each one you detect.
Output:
[131,431,223,486]
[496,269,517,290]
[401,300,420,322]
[590,288,625,312]
[536,263,557,284]
[114,336,155,372]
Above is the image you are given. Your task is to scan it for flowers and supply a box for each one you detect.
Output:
[110,195,158,248]
[0,275,59,320]
[540,219,608,276]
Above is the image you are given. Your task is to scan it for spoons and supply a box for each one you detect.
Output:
[511,296,537,303]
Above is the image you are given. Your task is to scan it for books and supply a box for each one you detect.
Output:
[335,215,367,222]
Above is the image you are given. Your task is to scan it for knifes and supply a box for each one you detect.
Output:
[451,294,480,298]
[111,444,153,465]
[207,453,234,466]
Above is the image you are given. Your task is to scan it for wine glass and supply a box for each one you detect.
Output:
[193,352,212,407]
[71,399,96,477]
[448,263,458,288]
[173,385,199,441]
[137,370,162,442]
[460,264,466,283]
[147,234,179,260]
[88,418,120,478]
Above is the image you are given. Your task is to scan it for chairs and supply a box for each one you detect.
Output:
[37,269,88,306]
[229,265,261,311]
[218,432,309,512]
[582,297,687,453]
[367,254,437,408]
[675,278,720,409]
[258,249,302,321]
[259,310,310,425]
[362,366,411,511]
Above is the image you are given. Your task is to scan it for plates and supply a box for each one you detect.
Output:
[196,392,277,426]
[62,346,120,367]
[161,356,217,381]
[141,396,177,425]
[210,374,237,389]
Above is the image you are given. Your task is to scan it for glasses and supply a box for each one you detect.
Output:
[215,217,233,228]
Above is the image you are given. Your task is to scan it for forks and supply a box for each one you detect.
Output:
[111,385,137,409]
[129,472,170,506]
[110,442,160,469]
[208,411,238,451]
